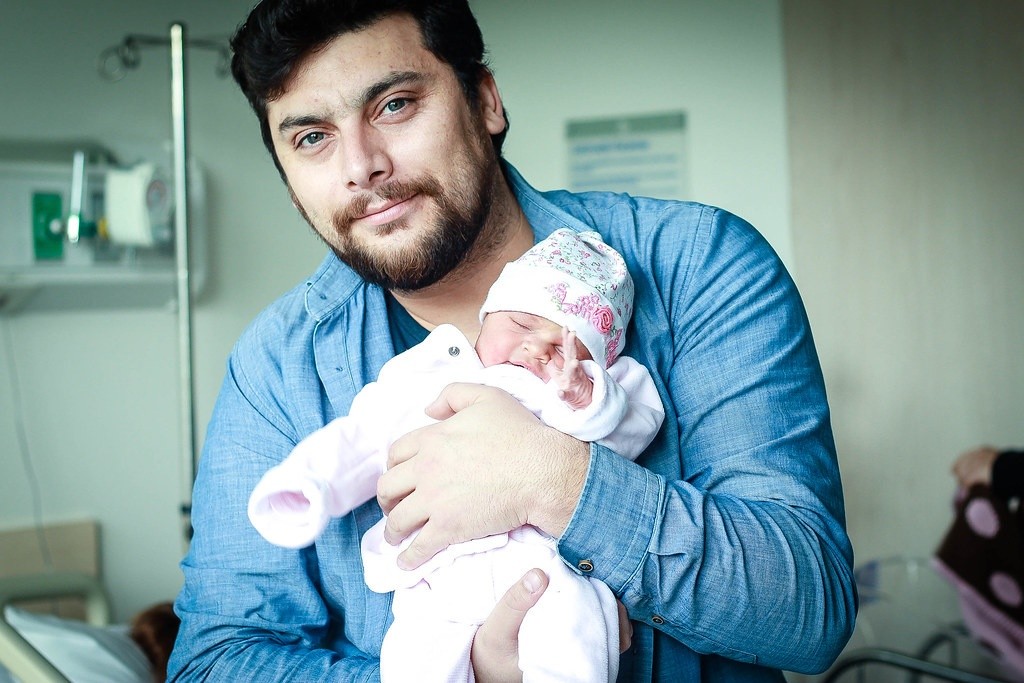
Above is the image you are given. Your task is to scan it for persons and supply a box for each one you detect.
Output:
[933,446,1024,683]
[246,228,665,683]
[165,0,858,682]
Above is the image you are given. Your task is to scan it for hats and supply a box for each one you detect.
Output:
[476,227,638,370]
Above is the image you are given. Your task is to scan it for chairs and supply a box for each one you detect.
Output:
[815,619,1024,683]
